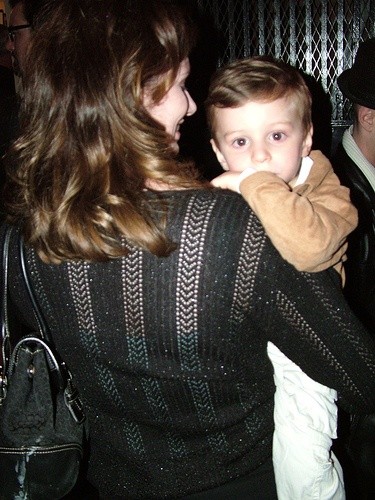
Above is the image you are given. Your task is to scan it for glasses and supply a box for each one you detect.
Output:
[4,23,33,42]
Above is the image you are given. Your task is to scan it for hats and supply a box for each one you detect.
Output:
[337,38,375,110]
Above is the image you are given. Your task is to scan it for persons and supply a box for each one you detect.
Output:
[0,1,375,500]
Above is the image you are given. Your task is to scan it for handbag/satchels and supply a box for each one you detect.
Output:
[0,226,88,500]
